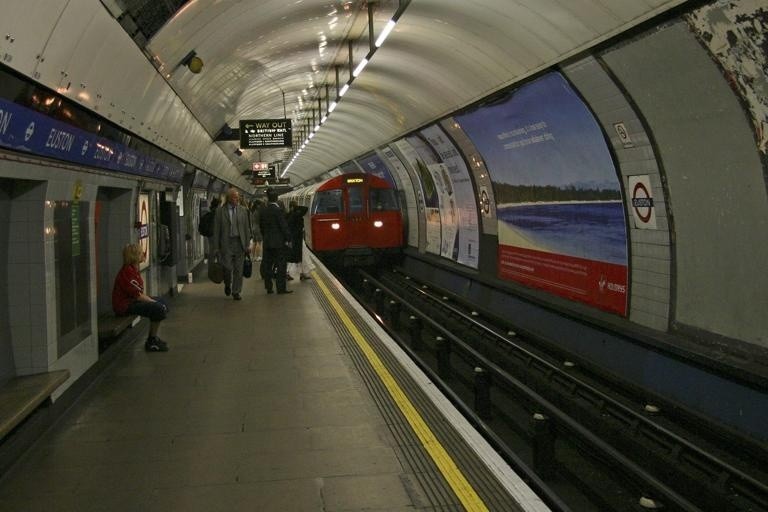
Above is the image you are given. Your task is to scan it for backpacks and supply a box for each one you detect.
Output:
[198,210,214,238]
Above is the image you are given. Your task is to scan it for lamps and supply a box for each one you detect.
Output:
[277,0,412,182]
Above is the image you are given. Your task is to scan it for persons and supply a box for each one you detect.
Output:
[111,243,169,351]
[204,187,317,294]
[210,188,251,301]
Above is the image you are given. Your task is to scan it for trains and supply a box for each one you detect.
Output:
[274,172,405,271]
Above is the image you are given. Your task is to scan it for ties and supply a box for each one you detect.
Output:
[231,207,236,236]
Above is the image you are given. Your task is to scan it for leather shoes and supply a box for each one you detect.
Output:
[224,285,232,297]
[277,289,294,294]
[268,289,274,294]
[232,293,242,301]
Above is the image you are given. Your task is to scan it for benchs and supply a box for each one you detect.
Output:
[97,311,141,341]
[1,369,71,445]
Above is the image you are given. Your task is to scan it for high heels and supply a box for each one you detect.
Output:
[286,274,294,281]
[299,273,312,280]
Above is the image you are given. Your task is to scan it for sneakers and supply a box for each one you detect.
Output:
[255,257,262,261]
[250,256,255,261]
[145,337,165,348]
[148,344,169,352]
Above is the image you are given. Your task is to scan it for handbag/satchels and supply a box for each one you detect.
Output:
[207,253,225,284]
[243,251,252,278]
